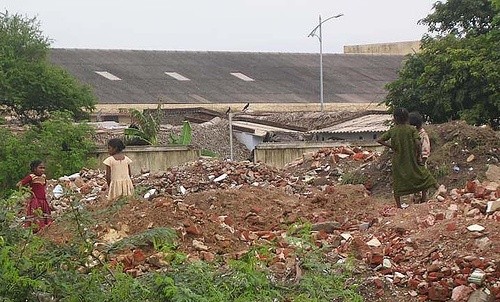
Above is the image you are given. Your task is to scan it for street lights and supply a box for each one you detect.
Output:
[307,13,346,111]
[225,102,250,161]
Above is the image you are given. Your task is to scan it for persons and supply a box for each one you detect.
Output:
[103,138,134,200]
[408,112,430,204]
[15,159,52,235]
[376,107,439,209]
